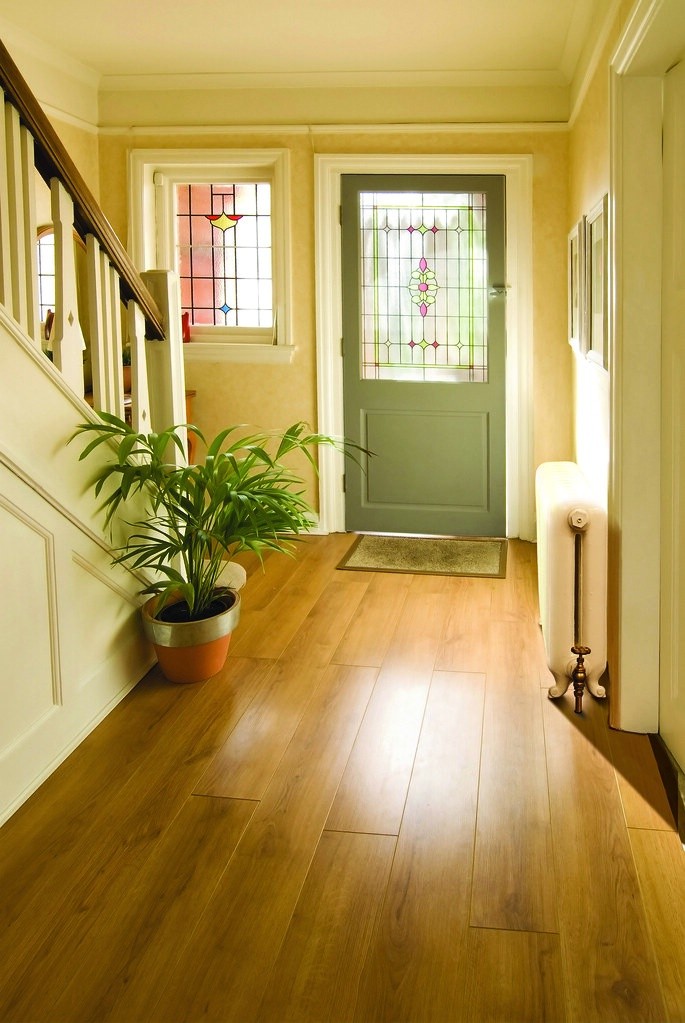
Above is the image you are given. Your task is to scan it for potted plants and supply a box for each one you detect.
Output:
[62,411,376,685]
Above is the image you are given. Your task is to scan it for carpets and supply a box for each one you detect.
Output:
[335,533,509,579]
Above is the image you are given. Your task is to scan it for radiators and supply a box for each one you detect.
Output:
[535,461,610,701]
